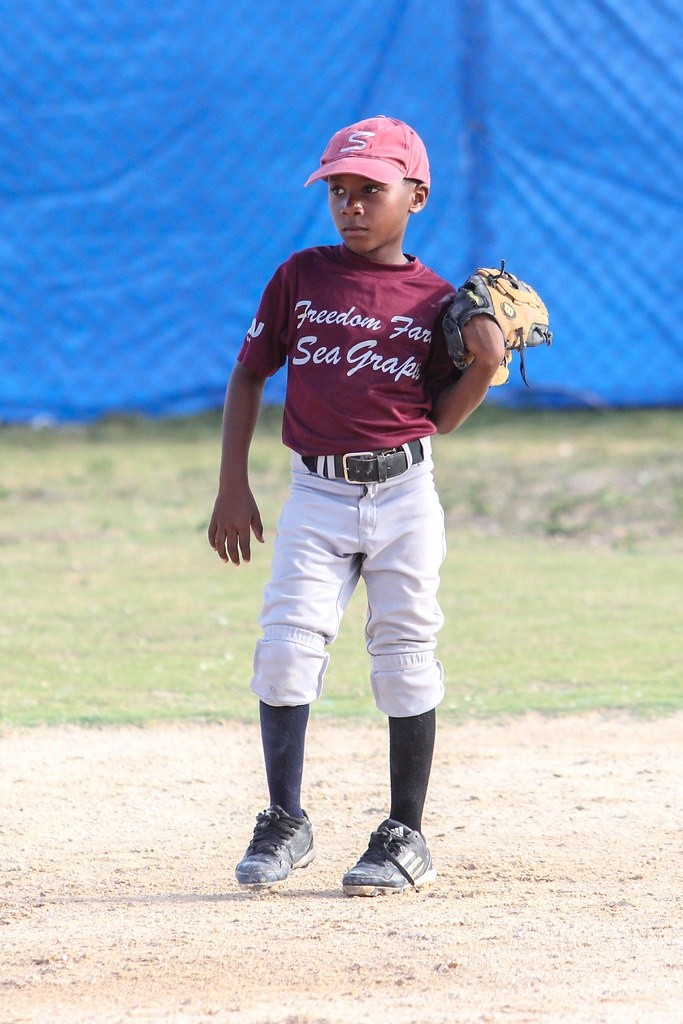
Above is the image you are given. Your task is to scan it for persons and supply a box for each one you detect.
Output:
[208,115,506,896]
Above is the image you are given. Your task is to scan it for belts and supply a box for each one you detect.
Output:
[302,439,424,485]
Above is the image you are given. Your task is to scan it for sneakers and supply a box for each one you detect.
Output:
[343,818,437,896]
[235,806,315,883]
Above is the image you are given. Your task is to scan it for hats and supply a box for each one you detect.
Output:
[304,115,430,188]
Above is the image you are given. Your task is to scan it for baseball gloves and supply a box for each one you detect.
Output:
[440,257,555,392]
